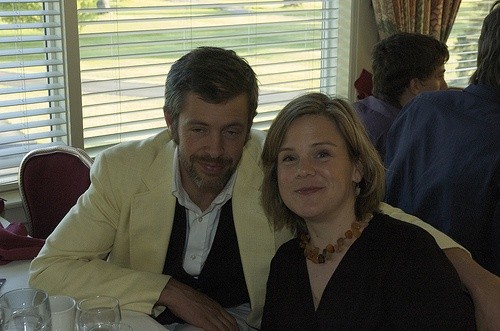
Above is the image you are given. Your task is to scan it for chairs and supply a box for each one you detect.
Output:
[17,146,94,240]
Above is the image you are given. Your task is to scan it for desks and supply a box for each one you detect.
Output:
[0,216,168,331]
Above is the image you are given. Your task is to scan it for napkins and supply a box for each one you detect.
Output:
[0,222,45,266]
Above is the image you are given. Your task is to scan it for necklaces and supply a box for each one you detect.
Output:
[297,214,371,264]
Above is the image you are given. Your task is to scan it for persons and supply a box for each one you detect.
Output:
[259,92,479,331]
[380,0,500,276]
[27,46,500,331]
[356,32,449,159]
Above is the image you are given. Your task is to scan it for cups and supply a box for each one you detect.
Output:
[75,296,121,331]
[50,295,75,331]
[0,287,50,331]
